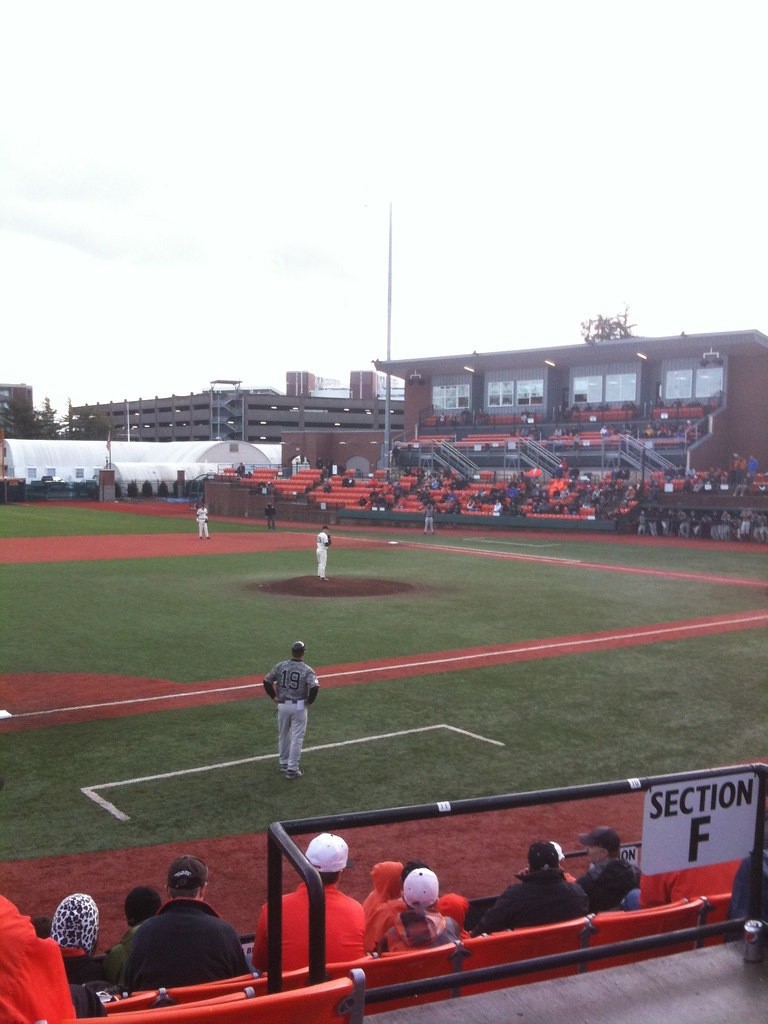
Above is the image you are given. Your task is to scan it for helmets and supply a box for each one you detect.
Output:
[292,641,306,658]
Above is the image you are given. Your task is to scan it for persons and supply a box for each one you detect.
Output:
[473,841,589,937]
[257,480,275,496]
[622,858,743,910]
[578,827,643,911]
[522,420,693,449]
[265,502,276,529]
[262,641,319,780]
[573,397,636,409]
[654,396,700,407]
[406,450,768,542]
[424,504,434,533]
[343,476,354,487]
[197,504,210,539]
[438,407,489,426]
[108,886,164,985]
[323,481,331,492]
[247,832,365,977]
[316,526,331,580]
[53,894,106,984]
[318,457,333,478]
[363,861,403,927]
[376,861,469,952]
[532,841,578,884]
[121,855,251,991]
[359,480,402,511]
[238,462,245,477]
[31,914,53,941]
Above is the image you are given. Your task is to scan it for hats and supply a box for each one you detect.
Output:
[402,868,439,908]
[323,525,329,529]
[167,855,208,889]
[125,887,162,926]
[578,825,620,851]
[401,859,431,879]
[527,841,565,867]
[305,833,349,872]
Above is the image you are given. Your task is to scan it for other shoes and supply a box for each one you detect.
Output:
[318,575,329,581]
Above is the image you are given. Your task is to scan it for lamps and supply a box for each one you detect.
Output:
[544,358,556,367]
[463,365,475,373]
[635,351,647,360]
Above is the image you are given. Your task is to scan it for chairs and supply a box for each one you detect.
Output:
[59,893,734,1024]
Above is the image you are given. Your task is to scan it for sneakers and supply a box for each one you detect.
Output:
[286,769,304,778]
[281,764,288,772]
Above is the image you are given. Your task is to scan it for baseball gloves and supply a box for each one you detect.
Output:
[204,519,208,523]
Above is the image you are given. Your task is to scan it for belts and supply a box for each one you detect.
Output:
[278,700,297,704]
